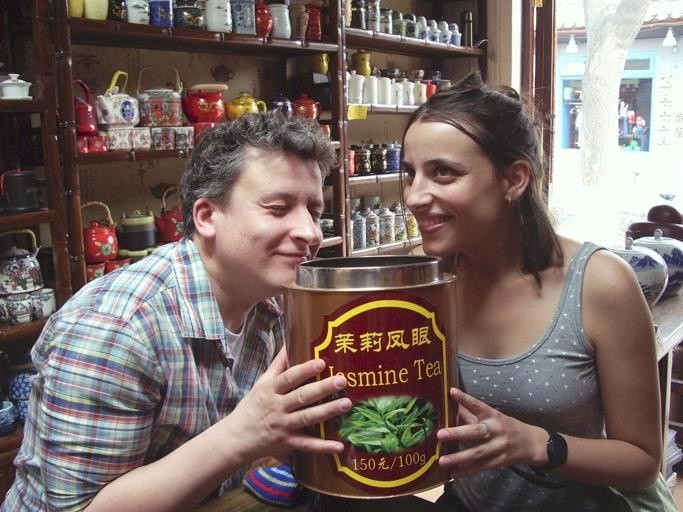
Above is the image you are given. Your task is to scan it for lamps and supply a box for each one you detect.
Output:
[562,35,582,54]
[659,26,677,48]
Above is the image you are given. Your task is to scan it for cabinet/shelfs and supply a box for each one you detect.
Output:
[62,0,343,290]
[339,3,487,258]
[0,0,80,507]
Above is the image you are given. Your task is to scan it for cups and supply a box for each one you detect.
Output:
[1,297,7,321]
[120,248,149,264]
[130,128,150,150]
[31,287,57,319]
[152,128,176,151]
[6,294,34,324]
[346,198,361,215]
[394,203,407,241]
[75,137,89,154]
[363,75,379,103]
[351,211,366,250]
[365,212,379,248]
[380,208,395,244]
[89,136,107,154]
[402,77,415,105]
[378,76,392,104]
[355,146,372,176]
[108,130,131,150]
[404,208,418,237]
[174,127,195,148]
[439,79,451,91]
[345,148,355,175]
[311,52,330,75]
[380,68,400,79]
[5,172,41,210]
[431,70,441,80]
[425,79,437,98]
[347,69,365,104]
[77,259,106,283]
[387,143,402,172]
[372,144,389,174]
[320,220,334,236]
[351,48,372,76]
[319,122,331,138]
[414,80,427,105]
[16,399,29,422]
[195,123,215,145]
[391,78,402,105]
[364,194,380,213]
[404,69,425,82]
[1,72,33,96]
[1,400,19,435]
[105,255,131,273]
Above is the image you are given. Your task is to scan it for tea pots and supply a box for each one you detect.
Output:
[293,92,322,120]
[82,199,120,263]
[118,206,157,250]
[224,91,267,122]
[135,64,186,127]
[74,80,100,136]
[0,229,45,294]
[182,84,228,121]
[94,70,140,128]
[9,372,42,402]
[154,183,184,242]
[266,91,296,119]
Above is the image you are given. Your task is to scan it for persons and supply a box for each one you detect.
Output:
[398,71,661,512]
[0,114,352,512]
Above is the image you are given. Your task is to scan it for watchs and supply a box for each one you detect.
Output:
[531,425,569,473]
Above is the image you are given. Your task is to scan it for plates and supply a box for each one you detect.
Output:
[0,94,35,101]
